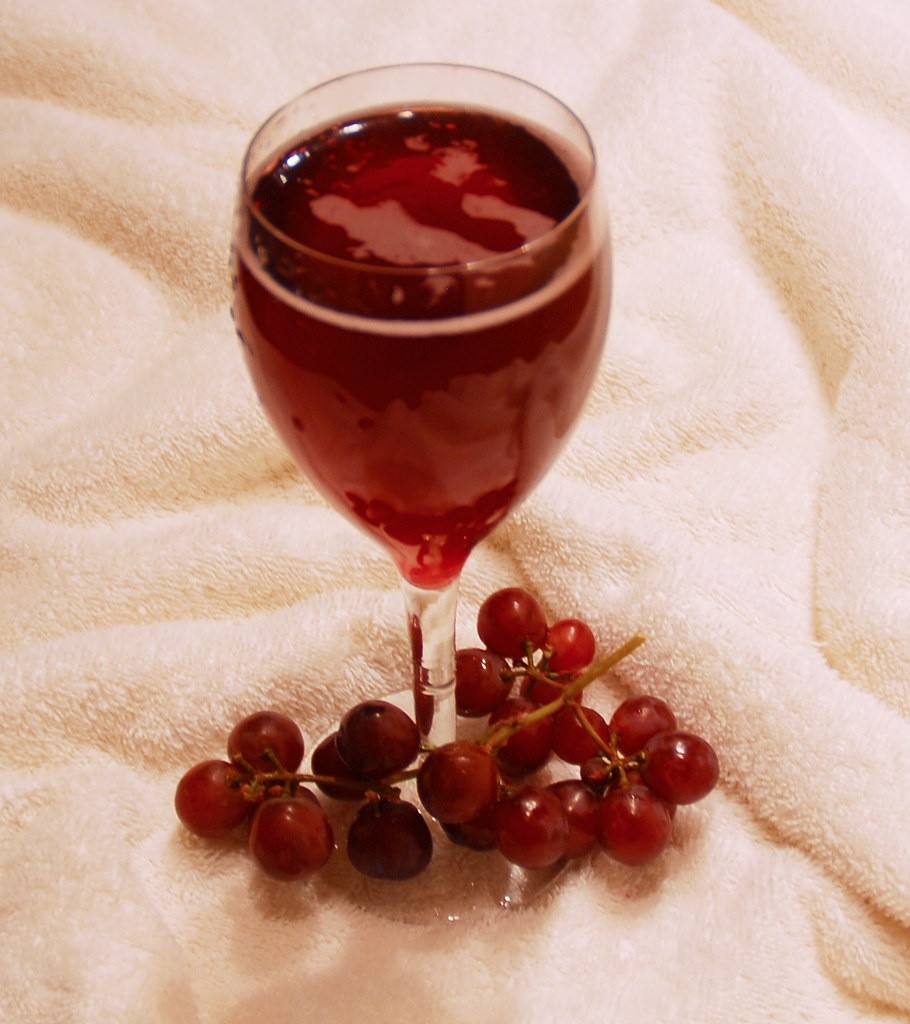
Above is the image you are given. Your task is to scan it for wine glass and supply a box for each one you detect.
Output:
[232,64,611,930]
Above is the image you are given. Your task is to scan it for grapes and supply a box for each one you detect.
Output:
[171,588,721,881]
